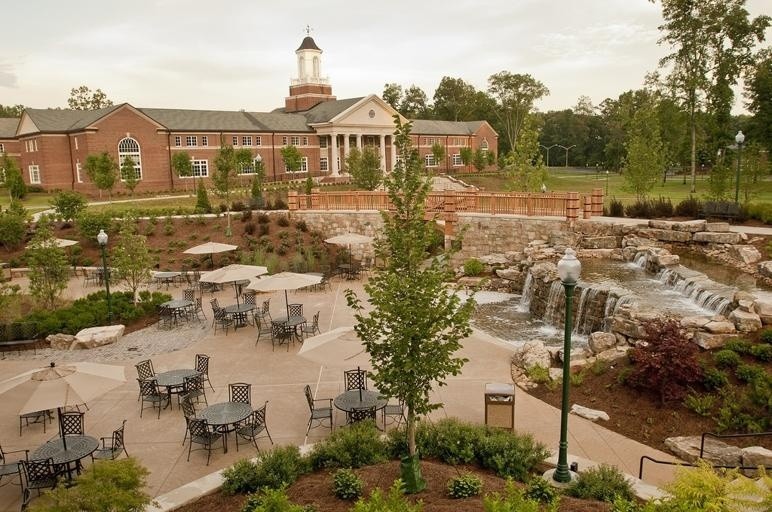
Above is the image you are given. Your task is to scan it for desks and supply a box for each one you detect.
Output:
[333,390,388,424]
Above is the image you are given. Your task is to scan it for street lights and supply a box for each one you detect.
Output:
[558,144,577,167]
[595,162,600,184]
[701,163,704,181]
[96,227,113,325]
[538,142,557,166]
[190,160,198,195]
[552,248,580,482]
[733,127,746,202]
[605,170,610,197]
[254,152,264,196]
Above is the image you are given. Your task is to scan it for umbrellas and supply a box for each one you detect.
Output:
[184,242,238,267]
[297,326,391,403]
[199,263,269,310]
[324,230,374,278]
[26,238,79,251]
[0,362,128,451]
[246,272,323,322]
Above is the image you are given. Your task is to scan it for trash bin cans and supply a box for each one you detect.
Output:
[485,382,515,434]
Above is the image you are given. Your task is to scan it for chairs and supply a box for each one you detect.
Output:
[154,267,223,331]
[290,284,324,294]
[304,385,334,436]
[1,409,129,512]
[80,266,110,286]
[347,406,377,425]
[0,255,406,512]
[1,322,43,359]
[211,291,270,336]
[336,259,374,282]
[344,370,367,421]
[252,302,322,352]
[381,395,406,431]
[180,382,274,466]
[134,350,215,419]
